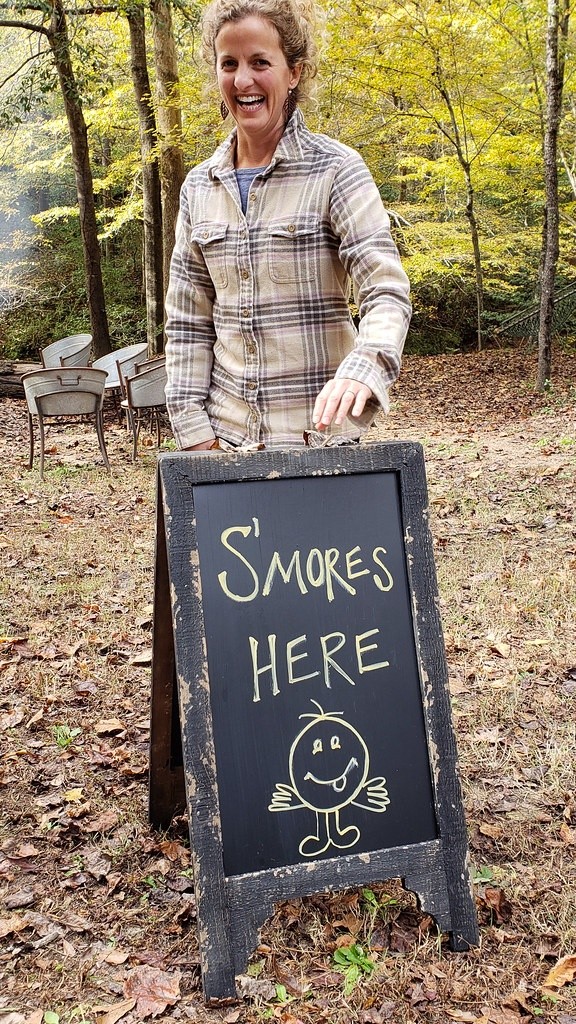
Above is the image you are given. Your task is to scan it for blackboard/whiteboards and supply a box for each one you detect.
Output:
[156,443,472,897]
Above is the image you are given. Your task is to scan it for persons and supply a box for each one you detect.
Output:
[164,0,413,451]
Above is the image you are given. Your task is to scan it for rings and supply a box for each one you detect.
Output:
[346,390,357,397]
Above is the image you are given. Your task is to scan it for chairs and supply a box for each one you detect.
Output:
[20,367,111,479]
[120,356,168,463]
[39,333,94,421]
[87,343,170,428]
